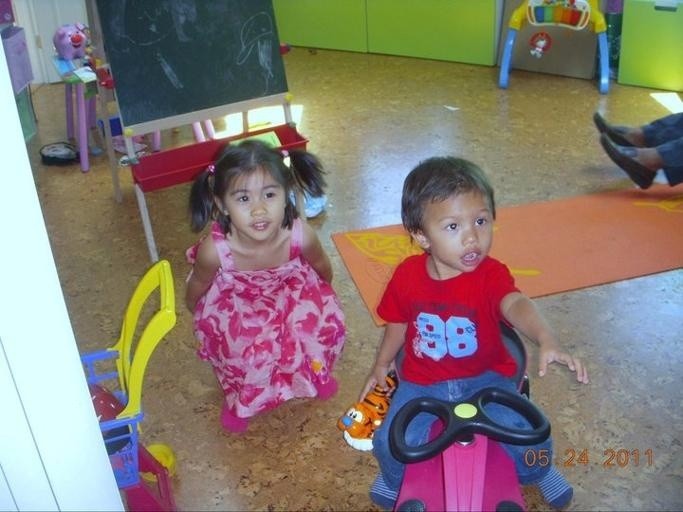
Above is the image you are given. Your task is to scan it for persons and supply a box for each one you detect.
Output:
[184,137,346,431]
[355,152,589,511]
[591,110,683,190]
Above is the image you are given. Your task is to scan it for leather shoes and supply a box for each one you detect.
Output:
[599,133,658,189]
[591,112,639,148]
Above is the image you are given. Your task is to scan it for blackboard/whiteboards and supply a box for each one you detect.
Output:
[95,0,291,138]
